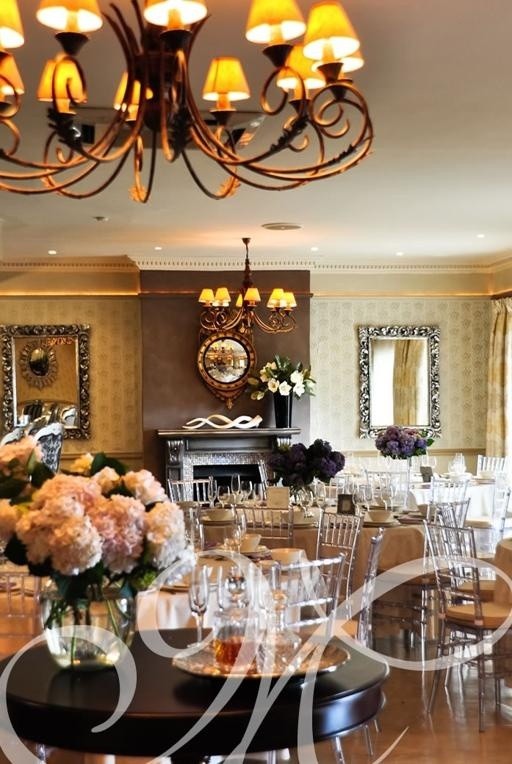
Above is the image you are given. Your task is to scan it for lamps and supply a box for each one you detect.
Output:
[0,0,378,202]
[193,237,299,337]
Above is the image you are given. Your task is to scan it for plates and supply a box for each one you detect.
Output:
[407,475,496,484]
[172,639,350,683]
[193,516,440,529]
[1,571,234,598]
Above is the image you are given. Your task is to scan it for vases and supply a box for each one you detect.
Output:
[272,388,291,427]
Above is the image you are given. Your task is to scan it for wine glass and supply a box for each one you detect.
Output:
[183,505,246,555]
[352,487,366,515]
[347,447,466,474]
[217,473,252,506]
[185,561,290,640]
[299,492,315,518]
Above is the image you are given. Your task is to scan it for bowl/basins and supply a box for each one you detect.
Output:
[287,511,314,526]
[240,532,263,551]
[365,510,393,524]
[419,505,440,516]
[206,509,229,521]
[270,545,302,565]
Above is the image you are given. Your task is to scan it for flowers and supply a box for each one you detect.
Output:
[245,353,315,406]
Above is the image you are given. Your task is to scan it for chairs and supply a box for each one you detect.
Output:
[0,422,512,764]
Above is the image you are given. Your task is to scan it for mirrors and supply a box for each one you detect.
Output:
[18,339,58,389]
[356,322,444,440]
[0,322,91,439]
